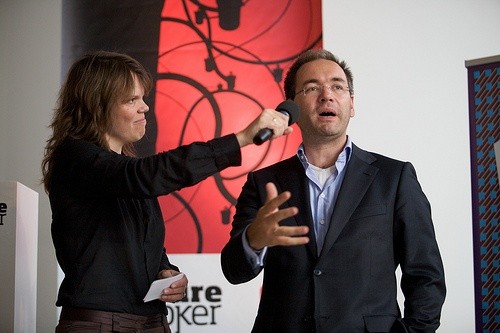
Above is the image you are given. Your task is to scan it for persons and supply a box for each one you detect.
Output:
[40,51,299,333]
[220,48,447,333]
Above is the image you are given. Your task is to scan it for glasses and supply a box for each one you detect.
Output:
[293,84,351,97]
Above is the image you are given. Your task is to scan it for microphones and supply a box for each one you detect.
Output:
[253,100,300,145]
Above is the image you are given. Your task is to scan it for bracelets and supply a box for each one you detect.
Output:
[246,225,264,254]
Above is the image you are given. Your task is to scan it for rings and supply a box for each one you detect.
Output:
[272,117,277,122]
[183,292,187,298]
